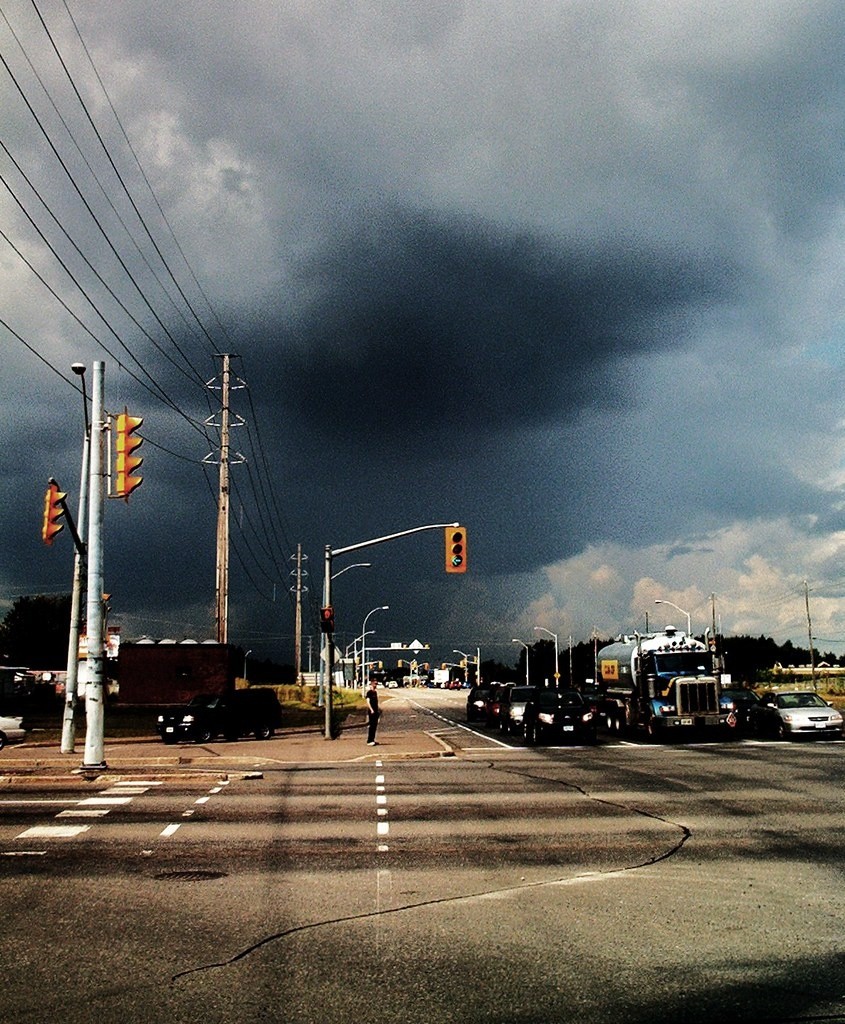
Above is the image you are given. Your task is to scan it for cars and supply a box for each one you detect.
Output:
[427,680,471,690]
[0,714,26,751]
[761,691,844,739]
[375,682,385,690]
[388,681,398,689]
[721,686,782,737]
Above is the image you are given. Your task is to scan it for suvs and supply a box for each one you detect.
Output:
[466,681,604,747]
[155,688,284,745]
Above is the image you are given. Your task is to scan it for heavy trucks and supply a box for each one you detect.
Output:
[596,625,737,744]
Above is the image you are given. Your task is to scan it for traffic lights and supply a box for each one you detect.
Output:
[115,414,145,499]
[43,486,68,546]
[319,608,335,633]
[100,592,112,613]
[446,527,466,573]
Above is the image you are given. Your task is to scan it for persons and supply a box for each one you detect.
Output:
[365,680,379,746]
[383,676,387,686]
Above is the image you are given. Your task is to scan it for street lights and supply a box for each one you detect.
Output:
[59,362,91,754]
[345,605,389,699]
[533,627,559,687]
[655,599,691,637]
[512,639,529,685]
[317,563,372,708]
[453,650,468,682]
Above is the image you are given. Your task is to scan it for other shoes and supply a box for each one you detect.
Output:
[367,741,377,746]
